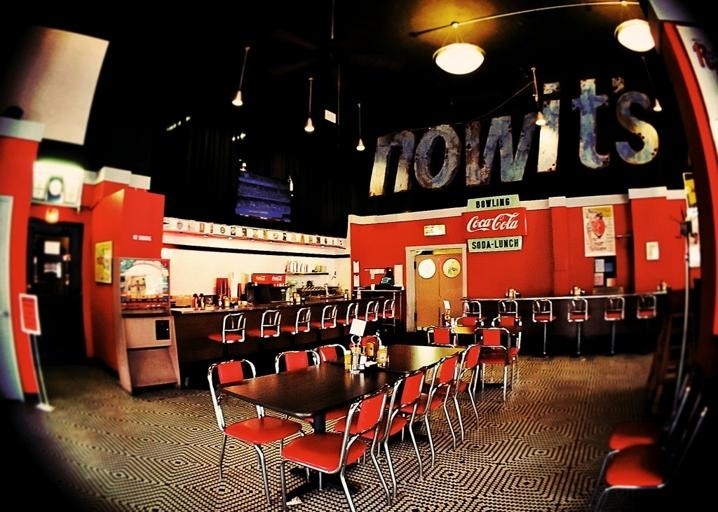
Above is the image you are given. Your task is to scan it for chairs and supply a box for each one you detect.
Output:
[246,310,281,338]
[207,313,246,343]
[312,343,347,364]
[604,296,625,356]
[462,300,487,320]
[274,350,349,456]
[588,394,708,511]
[473,327,513,401]
[337,303,358,326]
[358,301,379,322]
[609,373,692,450]
[281,307,311,335]
[498,300,522,321]
[361,335,382,347]
[532,298,557,358]
[567,297,592,357]
[280,383,391,511]
[425,344,481,442]
[427,328,458,348]
[207,359,310,505]
[387,352,458,465]
[636,295,656,353]
[311,304,337,329]
[335,370,424,497]
[454,317,484,328]
[378,299,395,319]
[491,317,521,389]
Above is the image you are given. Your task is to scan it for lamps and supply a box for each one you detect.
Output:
[641,55,662,112]
[432,22,487,75]
[232,48,250,106]
[530,67,546,126]
[613,2,655,52]
[304,78,315,132]
[356,103,366,151]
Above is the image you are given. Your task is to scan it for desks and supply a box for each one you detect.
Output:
[222,362,407,500]
[324,344,465,441]
